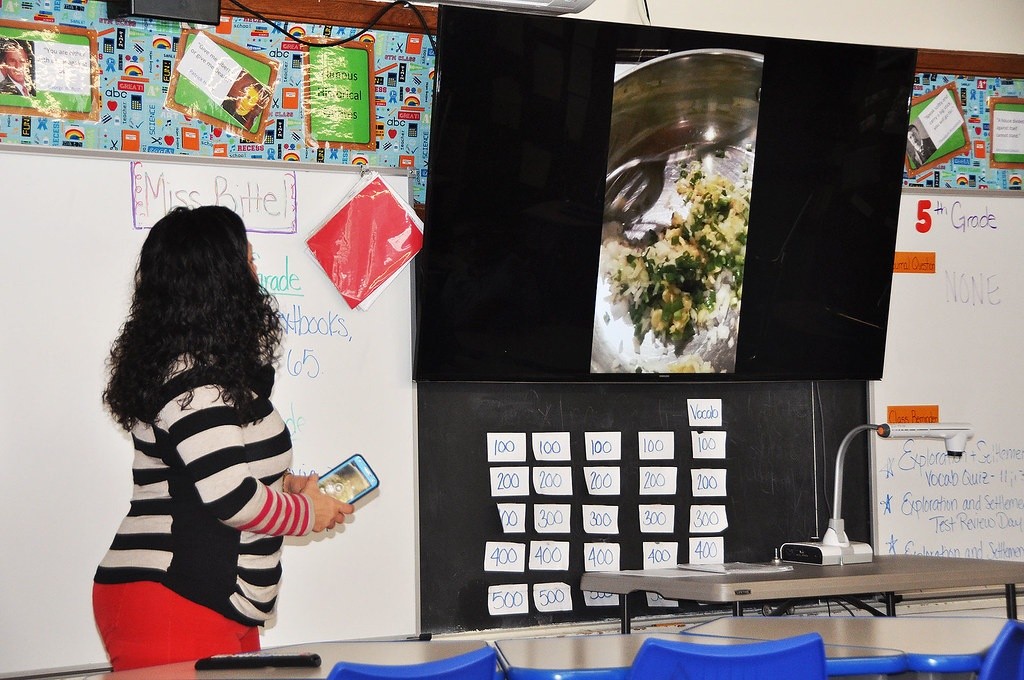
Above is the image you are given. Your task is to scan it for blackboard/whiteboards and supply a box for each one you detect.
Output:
[867,187,1024,599]
[0,143,424,680]
[414,381,878,637]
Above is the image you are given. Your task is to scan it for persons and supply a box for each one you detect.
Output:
[93,206,356,673]
[0,42,36,96]
[222,82,263,124]
[907,125,936,167]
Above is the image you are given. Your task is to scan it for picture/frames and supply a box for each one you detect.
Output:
[164,29,279,142]
[905,82,972,177]
[304,37,376,150]
[989,97,1024,168]
[0,19,101,121]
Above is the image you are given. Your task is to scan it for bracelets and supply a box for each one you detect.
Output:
[283,473,291,491]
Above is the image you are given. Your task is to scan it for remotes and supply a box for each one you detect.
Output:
[194,652,322,670]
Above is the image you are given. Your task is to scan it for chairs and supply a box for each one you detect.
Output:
[324,617,1024,680]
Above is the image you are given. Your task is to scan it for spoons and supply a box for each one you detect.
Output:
[602,48,763,373]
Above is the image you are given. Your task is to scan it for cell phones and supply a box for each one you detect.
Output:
[317,453,379,504]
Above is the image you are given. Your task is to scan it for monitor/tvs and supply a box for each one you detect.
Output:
[412,4,919,383]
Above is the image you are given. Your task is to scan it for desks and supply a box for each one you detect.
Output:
[579,555,1024,638]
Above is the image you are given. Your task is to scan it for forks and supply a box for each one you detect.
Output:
[605,120,757,231]
